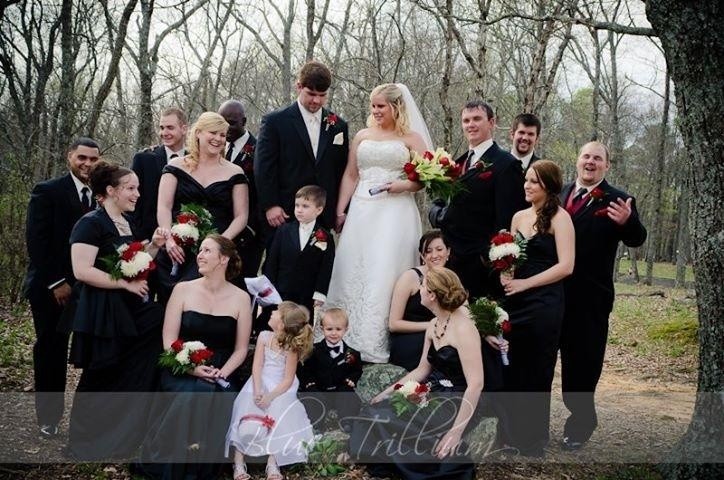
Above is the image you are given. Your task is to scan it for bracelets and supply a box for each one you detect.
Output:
[335,212,344,217]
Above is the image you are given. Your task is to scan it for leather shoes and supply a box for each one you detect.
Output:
[563,436,584,450]
[41,423,59,439]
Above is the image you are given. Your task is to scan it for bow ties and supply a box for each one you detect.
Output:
[327,346,341,354]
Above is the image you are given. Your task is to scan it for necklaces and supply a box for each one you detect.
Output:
[527,208,550,232]
[432,312,452,342]
[110,213,132,237]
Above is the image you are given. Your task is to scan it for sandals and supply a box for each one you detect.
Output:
[233,463,251,480]
[265,465,284,480]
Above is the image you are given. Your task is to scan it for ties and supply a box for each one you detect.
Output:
[81,187,90,207]
[573,187,588,204]
[463,150,476,172]
[225,142,236,162]
[171,154,179,159]
[310,117,320,149]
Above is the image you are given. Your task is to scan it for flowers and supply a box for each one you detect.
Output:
[586,189,612,204]
[242,144,259,163]
[155,339,233,389]
[369,147,469,201]
[247,271,278,310]
[471,299,516,365]
[164,205,219,276]
[322,107,339,131]
[392,380,443,420]
[100,244,151,304]
[341,348,357,368]
[474,159,492,176]
[485,229,529,278]
[308,228,327,246]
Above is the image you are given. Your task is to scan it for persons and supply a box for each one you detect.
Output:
[313,84,430,362]
[387,227,457,371]
[139,233,253,477]
[226,301,314,479]
[505,113,543,180]
[300,307,362,391]
[499,160,575,461]
[216,99,267,290]
[26,137,101,439]
[429,99,526,311]
[154,111,250,285]
[554,141,647,451]
[255,61,351,261]
[255,186,336,335]
[62,160,172,460]
[347,266,485,478]
[128,107,190,242]
